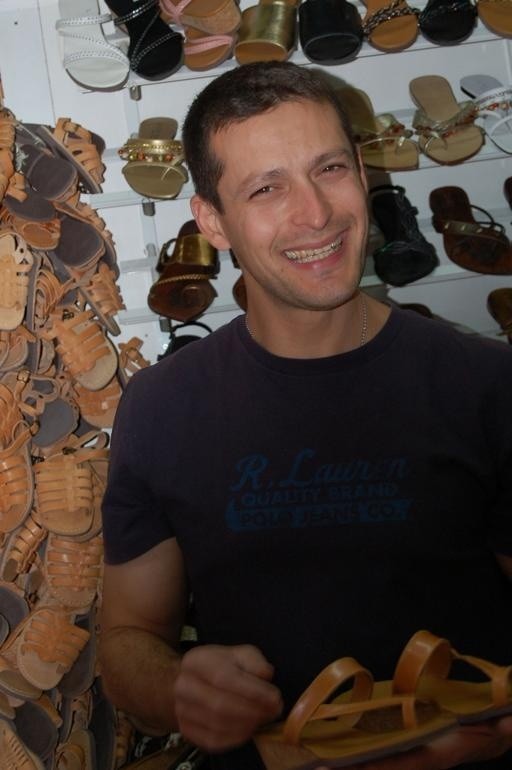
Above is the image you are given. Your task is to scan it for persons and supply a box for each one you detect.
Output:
[97,60,511,770]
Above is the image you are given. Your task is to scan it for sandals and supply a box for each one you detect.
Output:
[119,116,189,201]
[428,186,512,276]
[150,731,192,768]
[147,219,218,323]
[0,105,150,770]
[367,182,440,288]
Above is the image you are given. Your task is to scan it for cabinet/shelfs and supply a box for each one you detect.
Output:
[82,0,512,348]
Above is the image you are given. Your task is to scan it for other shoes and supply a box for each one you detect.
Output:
[486,287,512,348]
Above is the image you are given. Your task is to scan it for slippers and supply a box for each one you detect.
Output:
[253,655,459,769]
[331,629,511,725]
[53,0,512,94]
[335,74,512,169]
